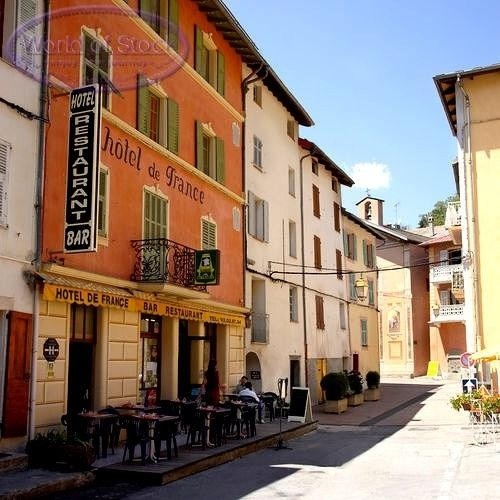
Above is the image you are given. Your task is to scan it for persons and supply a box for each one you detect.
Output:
[236,376,266,424]
[197,359,222,406]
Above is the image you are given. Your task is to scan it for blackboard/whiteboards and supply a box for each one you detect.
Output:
[288,387,309,423]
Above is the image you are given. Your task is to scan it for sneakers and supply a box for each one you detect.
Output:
[261,420,266,424]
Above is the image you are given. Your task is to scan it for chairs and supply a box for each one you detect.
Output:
[61,392,277,464]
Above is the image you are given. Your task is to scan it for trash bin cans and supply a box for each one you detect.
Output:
[474,373,479,381]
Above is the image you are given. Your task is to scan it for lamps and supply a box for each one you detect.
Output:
[354,273,369,301]
[432,301,439,317]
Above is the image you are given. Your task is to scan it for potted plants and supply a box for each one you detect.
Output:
[451,390,472,411]
[319,371,380,414]
